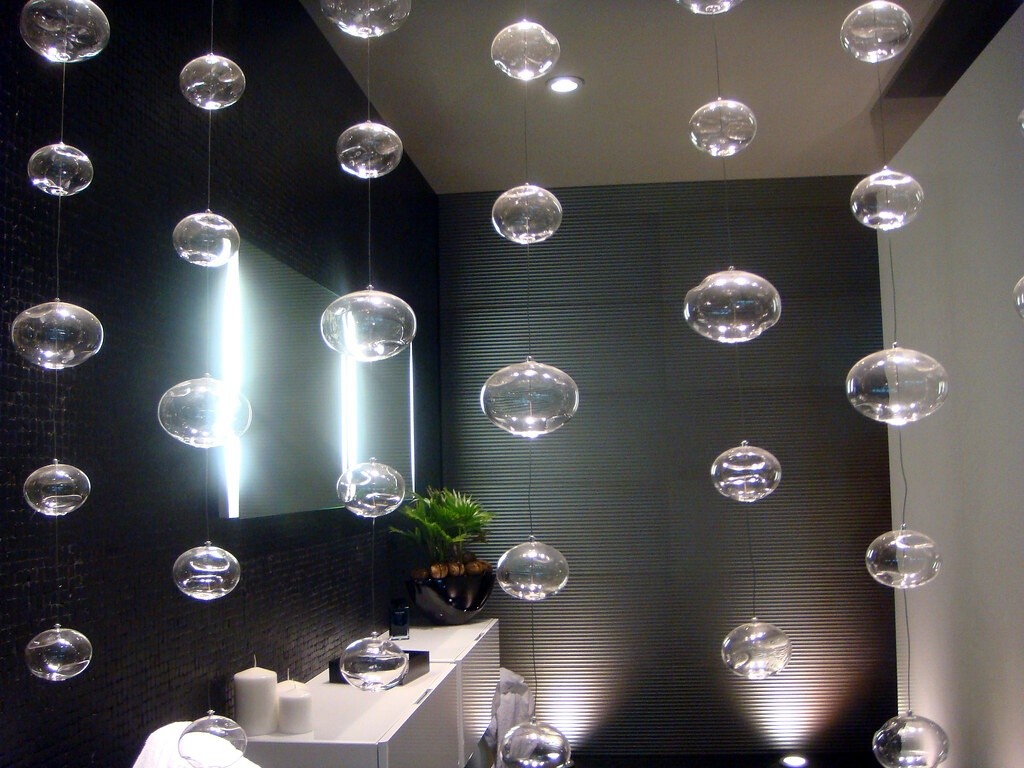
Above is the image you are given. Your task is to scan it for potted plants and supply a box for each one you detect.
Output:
[393,488,494,623]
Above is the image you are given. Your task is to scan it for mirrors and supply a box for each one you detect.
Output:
[219,238,416,519]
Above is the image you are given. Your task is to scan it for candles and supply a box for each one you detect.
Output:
[279,686,313,736]
[277,667,307,700]
[233,655,277,739]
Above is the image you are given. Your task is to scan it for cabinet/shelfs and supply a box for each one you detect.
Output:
[378,665,462,768]
[458,619,501,768]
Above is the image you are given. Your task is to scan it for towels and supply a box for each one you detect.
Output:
[484,666,535,768]
[131,721,262,768]
